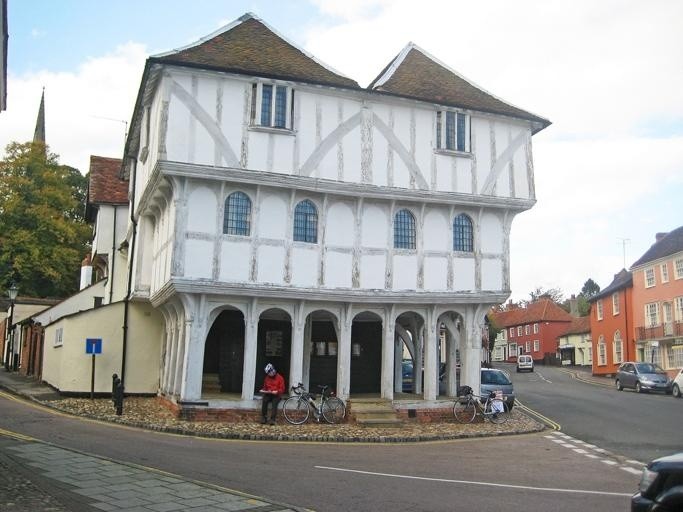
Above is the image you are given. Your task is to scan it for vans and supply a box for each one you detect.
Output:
[515,354,534,373]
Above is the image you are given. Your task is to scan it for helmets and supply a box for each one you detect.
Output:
[264,363,273,373]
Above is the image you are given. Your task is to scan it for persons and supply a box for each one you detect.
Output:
[258,363,285,425]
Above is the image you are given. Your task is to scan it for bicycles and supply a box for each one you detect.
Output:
[450,384,510,425]
[282,380,347,425]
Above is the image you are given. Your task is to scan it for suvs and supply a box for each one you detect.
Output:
[627,445,682,512]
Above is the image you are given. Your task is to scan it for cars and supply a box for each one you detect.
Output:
[401,360,515,412]
[614,360,672,393]
[670,365,683,398]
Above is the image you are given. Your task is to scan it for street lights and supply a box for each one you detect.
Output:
[3,283,19,372]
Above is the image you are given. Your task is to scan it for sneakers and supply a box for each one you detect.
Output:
[260,415,268,424]
[269,419,275,425]
